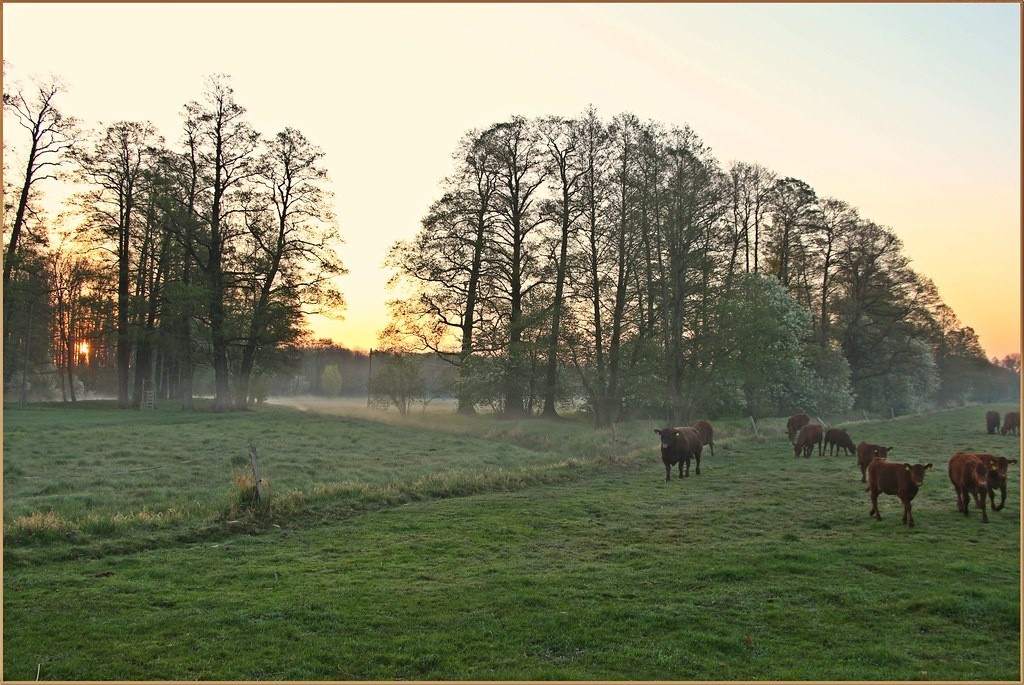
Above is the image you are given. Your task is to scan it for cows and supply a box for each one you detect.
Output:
[792,424,823,459]
[948,451,1017,524]
[986,410,1020,436]
[857,441,894,483]
[654,420,714,482]
[866,457,933,529]
[785,414,810,441]
[823,428,856,457]
